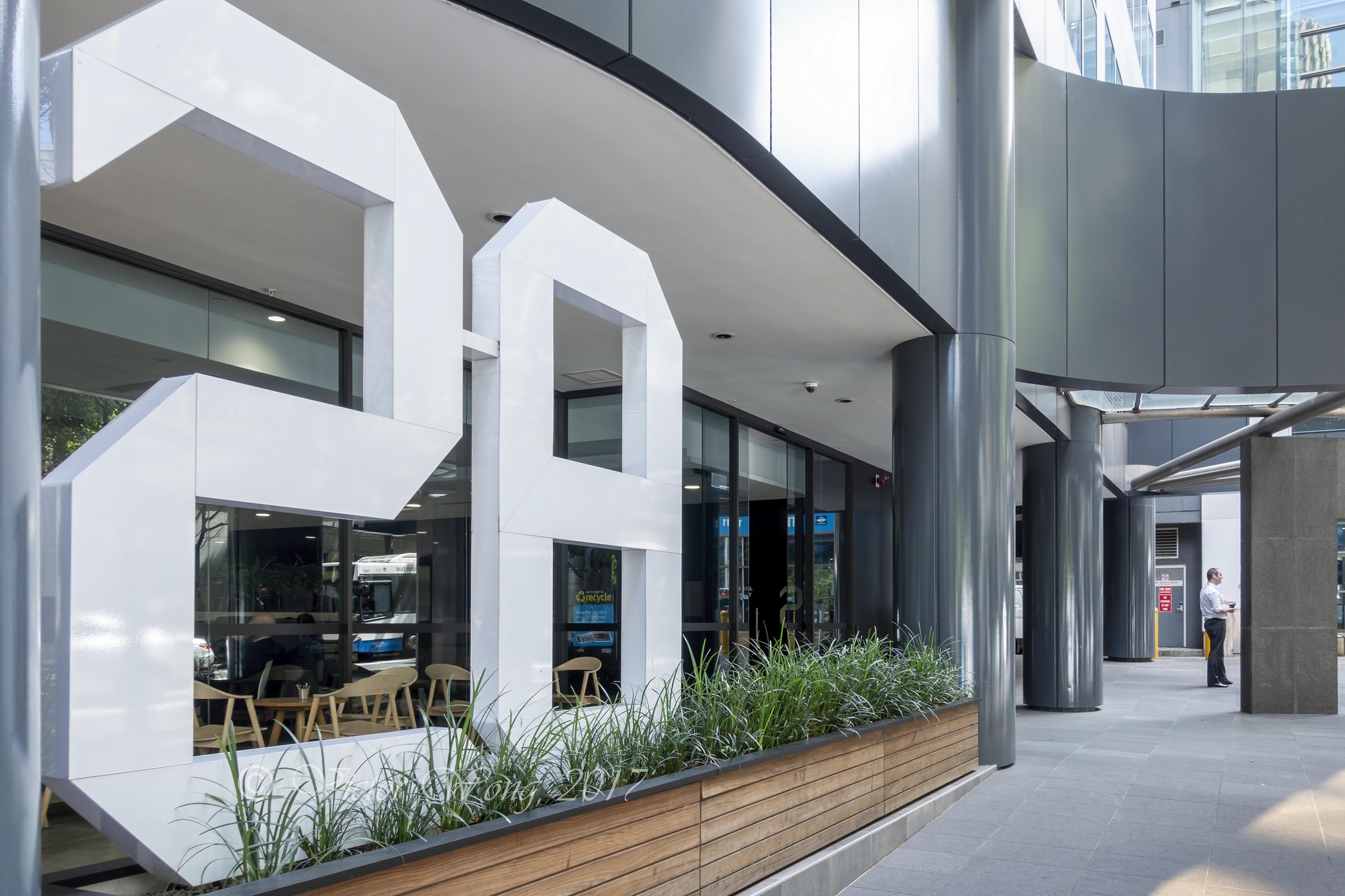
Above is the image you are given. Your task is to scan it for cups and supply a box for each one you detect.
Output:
[298,688,310,700]
[1229,604,1235,608]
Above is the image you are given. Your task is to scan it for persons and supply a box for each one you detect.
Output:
[1200,568,1237,687]
[286,613,324,695]
[356,592,371,612]
[199,614,288,727]
[250,588,272,612]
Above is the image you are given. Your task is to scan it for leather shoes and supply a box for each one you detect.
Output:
[1207,682,1228,687]
[1221,680,1233,684]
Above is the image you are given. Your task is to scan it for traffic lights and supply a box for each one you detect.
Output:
[719,588,729,599]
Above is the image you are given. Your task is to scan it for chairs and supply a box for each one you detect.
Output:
[424,663,471,726]
[193,666,420,757]
[552,656,602,709]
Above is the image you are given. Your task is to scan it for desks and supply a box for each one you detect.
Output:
[251,694,347,746]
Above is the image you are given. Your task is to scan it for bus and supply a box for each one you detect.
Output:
[322,552,616,680]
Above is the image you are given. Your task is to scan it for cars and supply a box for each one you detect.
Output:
[193,638,214,669]
